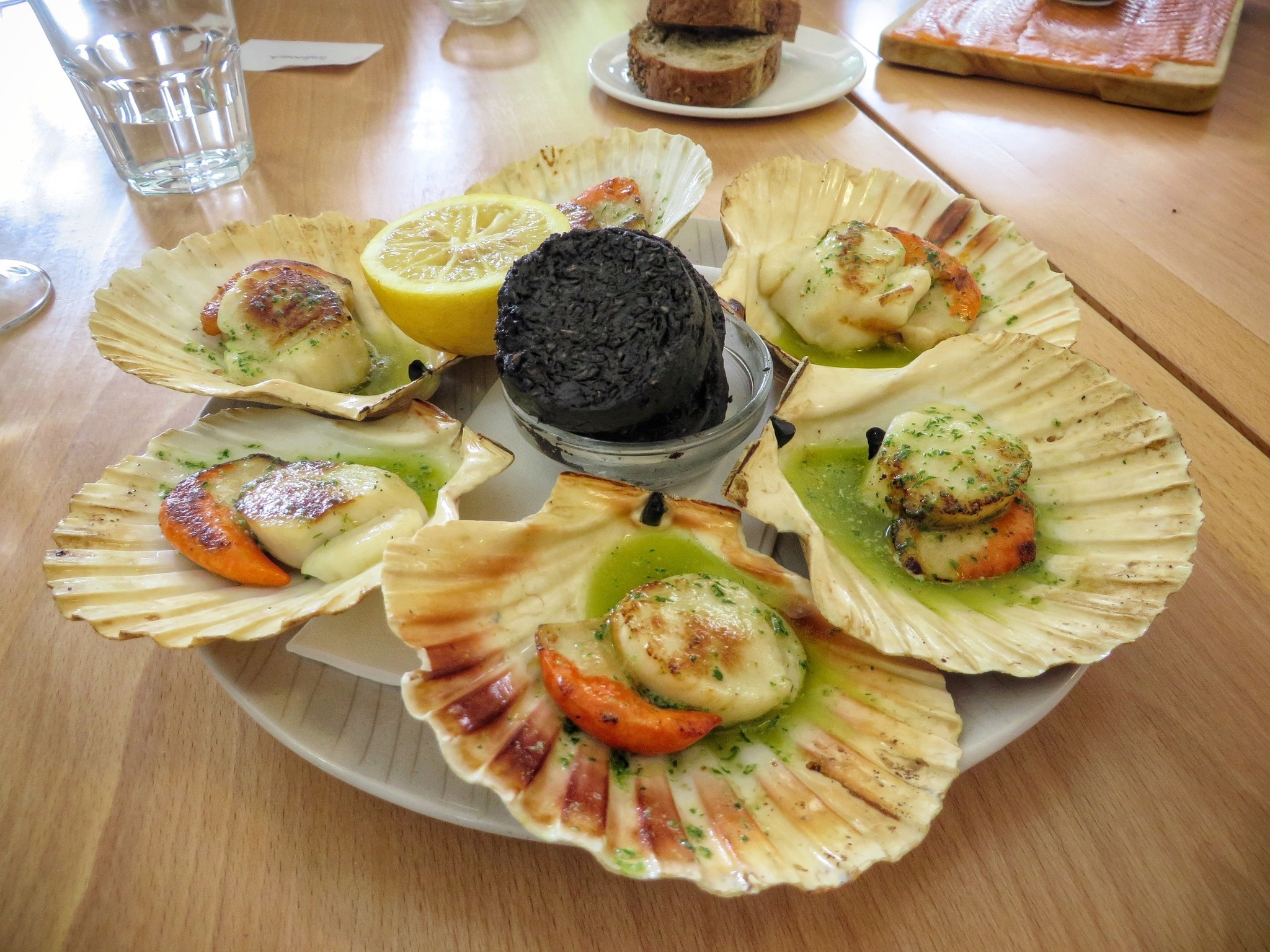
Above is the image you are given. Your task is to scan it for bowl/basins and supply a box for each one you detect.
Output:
[503,312,779,488]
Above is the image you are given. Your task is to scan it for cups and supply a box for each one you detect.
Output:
[440,1,527,25]
[30,1,255,197]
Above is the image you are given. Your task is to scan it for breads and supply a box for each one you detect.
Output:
[628,0,804,108]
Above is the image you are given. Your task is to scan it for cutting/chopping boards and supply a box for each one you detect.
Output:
[877,1,1245,110]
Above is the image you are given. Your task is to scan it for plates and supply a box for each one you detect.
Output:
[198,220,1094,841]
[587,23,868,118]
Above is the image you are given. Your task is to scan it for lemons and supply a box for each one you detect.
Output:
[360,194,572,356]
